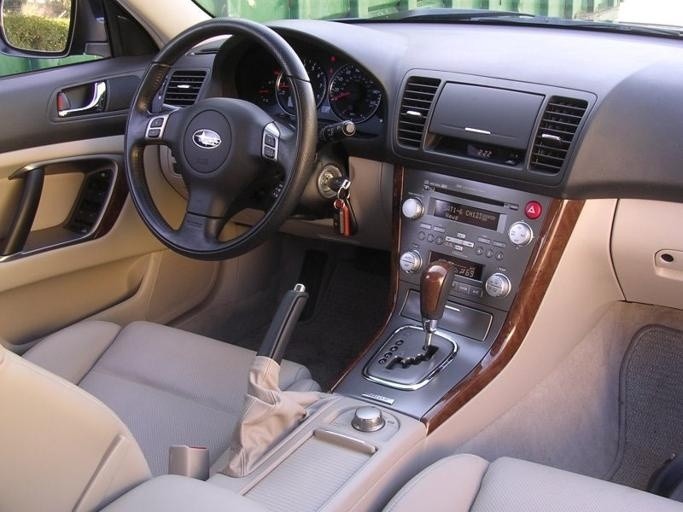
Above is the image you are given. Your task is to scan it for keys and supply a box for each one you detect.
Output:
[325,176,352,198]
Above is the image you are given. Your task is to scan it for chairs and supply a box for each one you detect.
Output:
[3,316,320,512]
[379,454,682,511]
